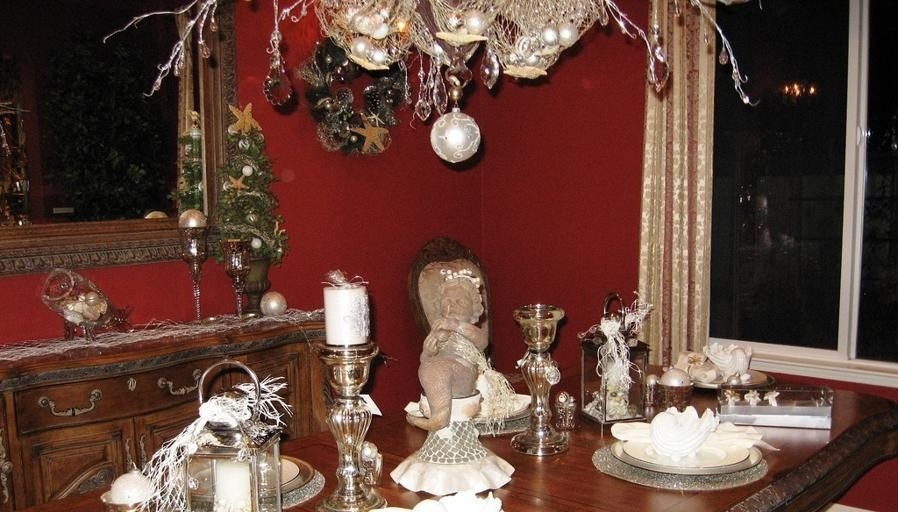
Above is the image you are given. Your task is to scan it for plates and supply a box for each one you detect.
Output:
[692,366,774,391]
[165,455,314,498]
[405,391,531,428]
[613,444,760,473]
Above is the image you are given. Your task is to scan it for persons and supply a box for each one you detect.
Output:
[389,275,515,497]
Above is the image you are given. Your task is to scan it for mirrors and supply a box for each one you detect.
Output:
[0,0,237,276]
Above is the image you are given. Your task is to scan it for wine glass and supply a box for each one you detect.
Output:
[180,228,250,323]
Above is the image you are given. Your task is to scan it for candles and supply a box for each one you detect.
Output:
[321,269,370,346]
[213,459,251,511]
[599,356,628,417]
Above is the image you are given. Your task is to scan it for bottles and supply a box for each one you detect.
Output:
[42,269,111,330]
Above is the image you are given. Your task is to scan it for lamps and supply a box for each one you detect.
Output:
[102,0,765,165]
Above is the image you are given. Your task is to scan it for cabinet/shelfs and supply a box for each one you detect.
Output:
[0,310,331,512]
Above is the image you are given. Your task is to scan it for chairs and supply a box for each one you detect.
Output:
[406,236,524,386]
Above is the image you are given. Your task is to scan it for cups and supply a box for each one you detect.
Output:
[99,491,151,512]
[655,381,694,413]
